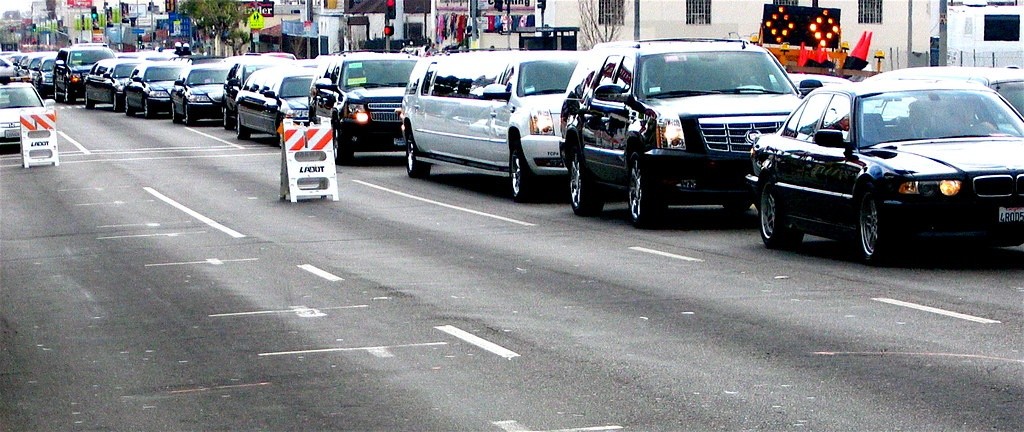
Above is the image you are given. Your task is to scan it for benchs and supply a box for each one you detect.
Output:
[886,100,937,141]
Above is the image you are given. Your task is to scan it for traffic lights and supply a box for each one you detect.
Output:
[138,34,142,44]
[386,0,396,19]
[537,0,546,8]
[91,6,97,19]
[385,26,394,36]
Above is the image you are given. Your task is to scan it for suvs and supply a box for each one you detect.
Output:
[307,48,429,167]
[392,43,588,204]
[556,35,822,232]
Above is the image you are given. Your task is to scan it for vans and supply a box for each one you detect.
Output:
[51,45,116,105]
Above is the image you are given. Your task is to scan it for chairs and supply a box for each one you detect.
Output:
[846,114,887,146]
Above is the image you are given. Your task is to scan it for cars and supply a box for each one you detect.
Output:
[219,56,293,131]
[0,45,58,145]
[169,62,236,126]
[81,58,146,113]
[233,63,317,150]
[745,74,1024,267]
[123,59,192,119]
[790,63,1024,122]
